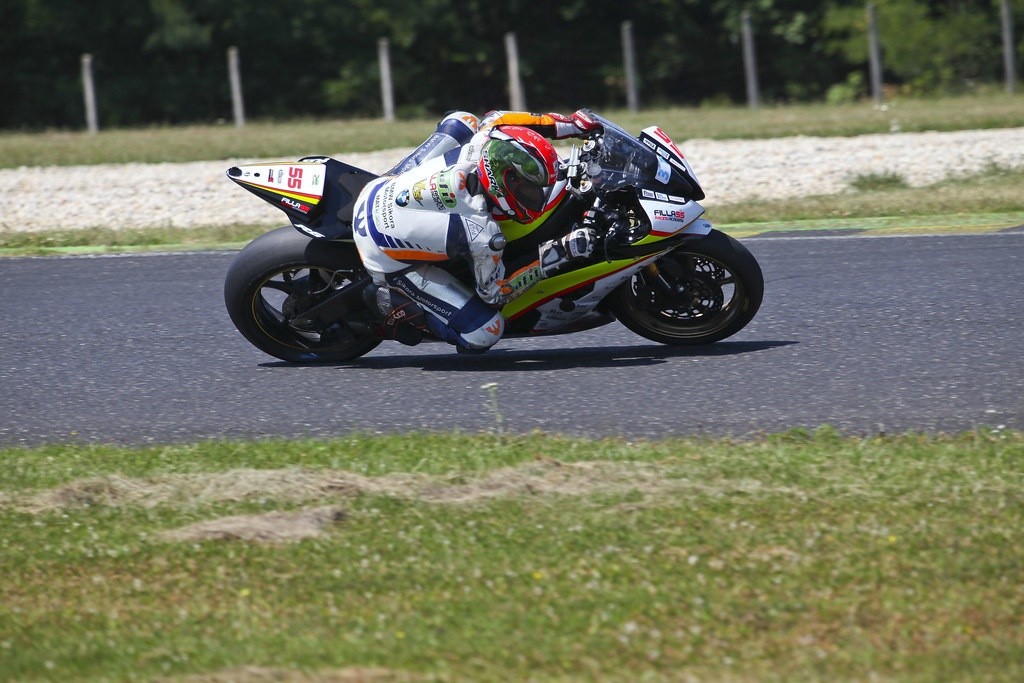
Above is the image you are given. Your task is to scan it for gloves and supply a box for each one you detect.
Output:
[546,107,604,140]
[538,227,598,278]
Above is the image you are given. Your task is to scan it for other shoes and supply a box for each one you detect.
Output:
[362,284,422,346]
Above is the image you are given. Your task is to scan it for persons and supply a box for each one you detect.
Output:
[352,108,606,355]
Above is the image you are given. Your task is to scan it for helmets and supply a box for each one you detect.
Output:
[478,126,557,225]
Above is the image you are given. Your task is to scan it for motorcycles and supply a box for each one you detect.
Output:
[223,109,765,365]
